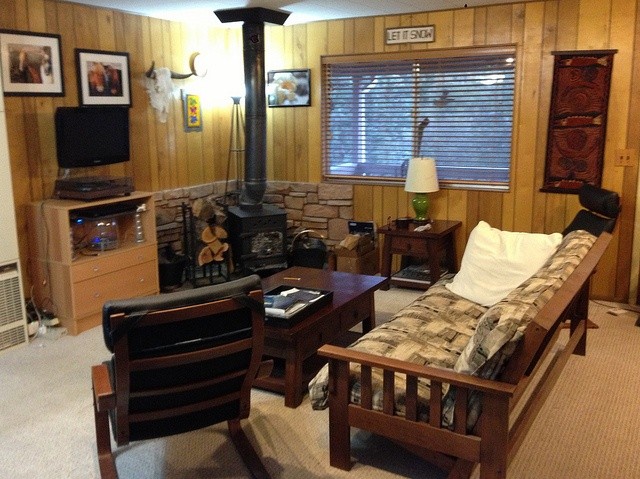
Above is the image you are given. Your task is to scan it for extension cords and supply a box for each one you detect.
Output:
[28,321,39,335]
[39,317,59,326]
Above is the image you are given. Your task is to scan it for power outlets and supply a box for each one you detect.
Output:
[615,149,632,166]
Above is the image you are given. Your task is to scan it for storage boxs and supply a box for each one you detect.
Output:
[337,249,381,275]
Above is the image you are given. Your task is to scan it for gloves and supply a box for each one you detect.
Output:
[413,223,432,232]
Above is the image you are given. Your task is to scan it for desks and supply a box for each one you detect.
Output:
[377,217,462,291]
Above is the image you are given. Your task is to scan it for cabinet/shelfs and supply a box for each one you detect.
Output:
[31,188,159,336]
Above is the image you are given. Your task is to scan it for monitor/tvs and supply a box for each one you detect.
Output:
[55,105,130,169]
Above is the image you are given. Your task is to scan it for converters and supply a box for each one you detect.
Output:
[37,325,47,334]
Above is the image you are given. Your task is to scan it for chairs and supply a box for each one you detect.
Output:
[562,183,622,234]
[91,275,271,479]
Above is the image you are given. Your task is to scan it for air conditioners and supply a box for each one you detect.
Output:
[0,88,28,349]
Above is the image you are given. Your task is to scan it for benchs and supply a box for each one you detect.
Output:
[308,230,614,479]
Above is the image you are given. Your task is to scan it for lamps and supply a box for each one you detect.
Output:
[403,157,441,221]
[223,77,246,199]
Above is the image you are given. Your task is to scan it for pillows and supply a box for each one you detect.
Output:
[444,220,562,306]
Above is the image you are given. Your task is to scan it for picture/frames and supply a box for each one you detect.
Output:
[268,68,312,107]
[0,28,65,97]
[75,46,134,106]
